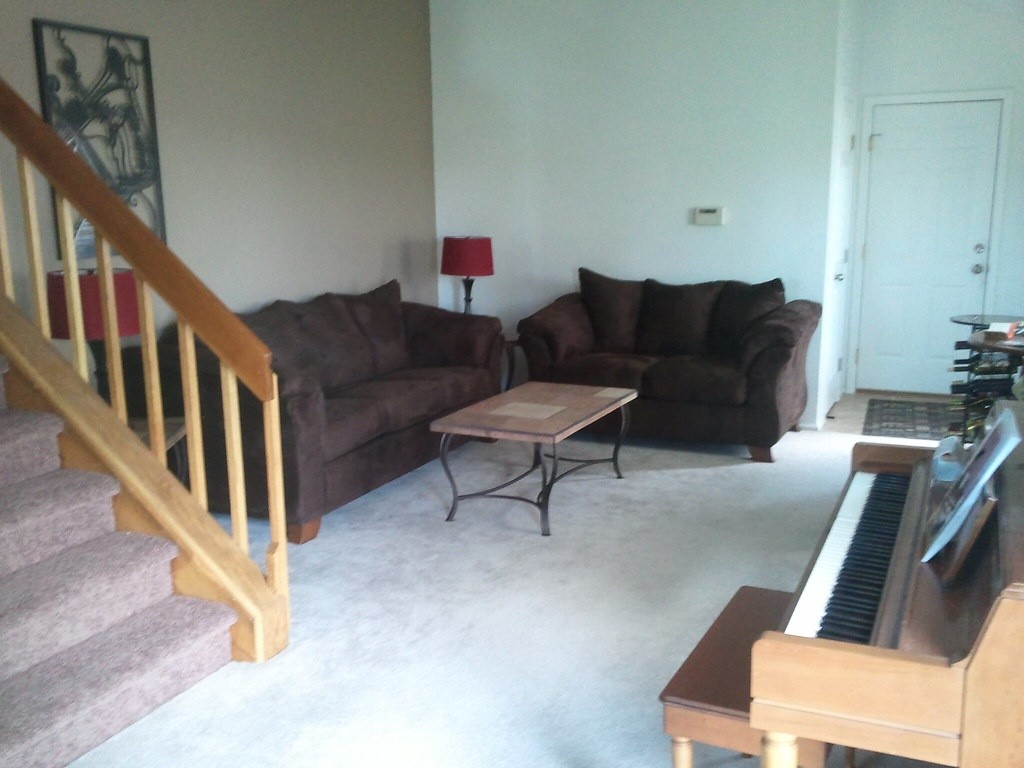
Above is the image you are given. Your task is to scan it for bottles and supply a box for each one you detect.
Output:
[947,335,1024,441]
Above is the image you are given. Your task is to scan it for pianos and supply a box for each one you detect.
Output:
[749,399,1023,767]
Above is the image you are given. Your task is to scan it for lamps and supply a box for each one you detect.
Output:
[46,268,139,405]
[439,237,496,314]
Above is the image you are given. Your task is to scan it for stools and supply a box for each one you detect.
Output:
[657,585,855,768]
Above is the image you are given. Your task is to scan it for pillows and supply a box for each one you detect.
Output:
[578,266,645,354]
[706,278,786,365]
[290,292,379,386]
[637,278,724,357]
[339,278,418,375]
[236,300,343,397]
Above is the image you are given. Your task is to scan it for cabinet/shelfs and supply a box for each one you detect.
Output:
[951,314,1024,444]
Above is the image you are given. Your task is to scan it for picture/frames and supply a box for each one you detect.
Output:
[31,18,167,264]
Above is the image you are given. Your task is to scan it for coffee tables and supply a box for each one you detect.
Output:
[430,381,639,535]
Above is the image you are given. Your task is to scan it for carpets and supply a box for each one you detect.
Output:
[861,398,964,441]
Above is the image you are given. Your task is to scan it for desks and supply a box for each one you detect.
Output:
[968,330,1024,356]
[128,415,206,484]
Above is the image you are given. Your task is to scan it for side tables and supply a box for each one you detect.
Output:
[503,340,522,391]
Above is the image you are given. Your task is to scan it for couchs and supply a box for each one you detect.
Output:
[517,292,824,465]
[157,301,512,544]
